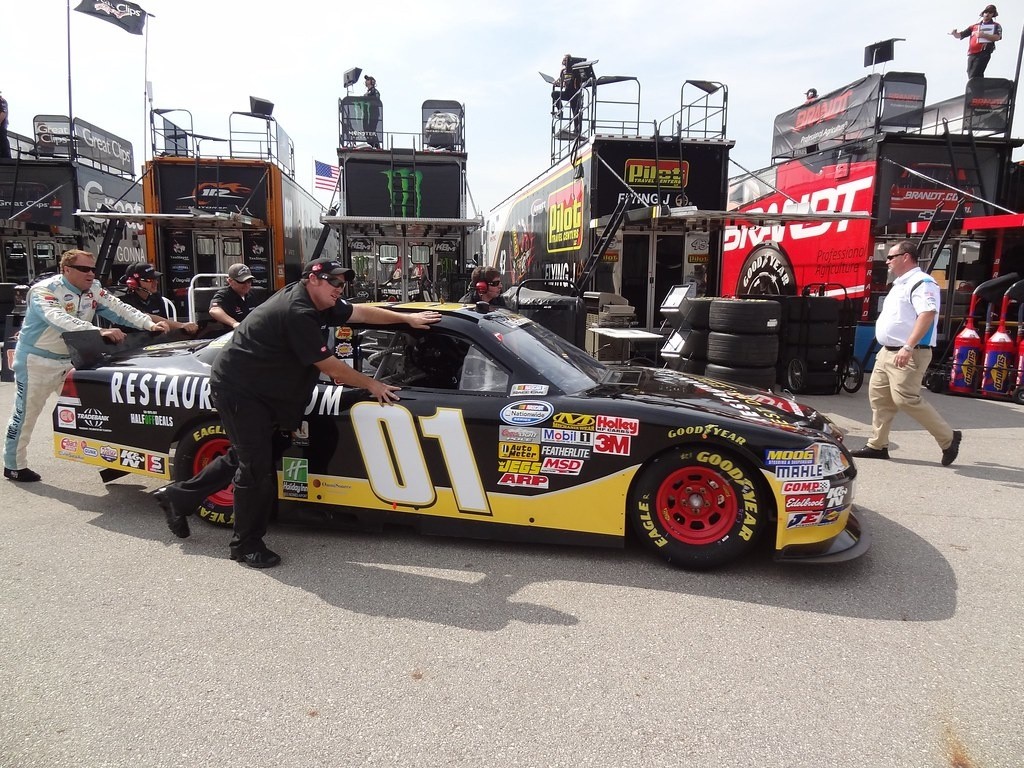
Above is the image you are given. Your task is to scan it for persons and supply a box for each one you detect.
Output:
[361,76,381,150]
[459,267,506,308]
[0,91,11,159]
[949,5,1004,80]
[200,263,266,340]
[154,260,443,567]
[114,261,198,335]
[4,248,170,482]
[848,240,962,467]
[550,57,582,136]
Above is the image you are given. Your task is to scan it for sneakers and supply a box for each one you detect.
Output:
[153,488,190,538]
[228,546,280,568]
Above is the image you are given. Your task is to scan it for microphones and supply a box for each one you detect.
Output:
[138,287,153,296]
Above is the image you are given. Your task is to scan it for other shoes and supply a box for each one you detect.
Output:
[941,430,962,466]
[850,444,890,459]
[4,467,41,482]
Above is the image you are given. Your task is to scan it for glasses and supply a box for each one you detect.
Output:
[319,274,347,289]
[236,277,252,284]
[488,280,500,287]
[887,252,907,260]
[139,276,160,282]
[68,265,97,274]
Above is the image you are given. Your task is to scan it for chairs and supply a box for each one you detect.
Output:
[404,336,459,389]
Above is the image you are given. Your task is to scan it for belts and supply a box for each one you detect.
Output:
[884,343,929,351]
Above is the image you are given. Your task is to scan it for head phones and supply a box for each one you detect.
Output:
[808,88,814,98]
[126,261,139,290]
[476,267,488,294]
[980,6,999,17]
[372,78,375,85]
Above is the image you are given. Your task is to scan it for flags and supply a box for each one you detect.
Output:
[73,0,146,37]
[314,159,340,192]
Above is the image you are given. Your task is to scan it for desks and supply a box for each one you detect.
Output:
[589,327,665,367]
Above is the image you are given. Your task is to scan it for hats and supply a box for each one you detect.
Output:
[228,263,255,282]
[302,257,355,286]
[126,261,163,278]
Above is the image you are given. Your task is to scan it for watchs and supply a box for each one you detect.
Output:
[903,345,914,352]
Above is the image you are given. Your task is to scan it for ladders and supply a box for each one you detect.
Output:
[94,219,125,289]
[195,156,218,215]
[0,147,21,218]
[654,120,685,207]
[391,135,417,218]
[571,197,631,298]
[860,199,965,378]
[942,117,990,216]
